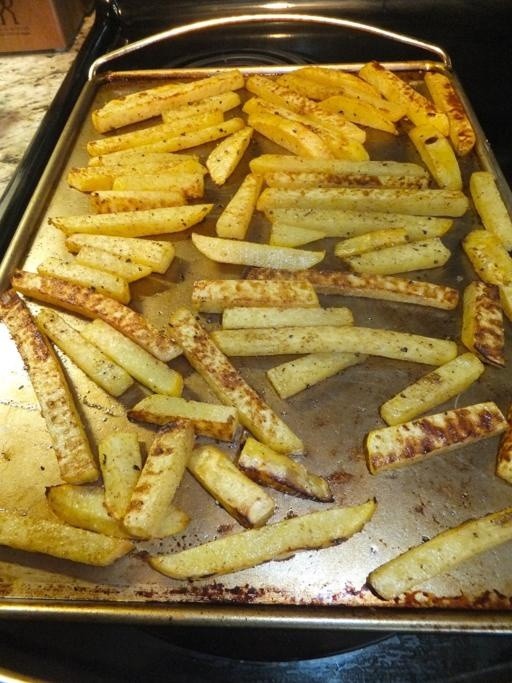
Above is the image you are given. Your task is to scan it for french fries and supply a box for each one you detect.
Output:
[1,59,512,602]
